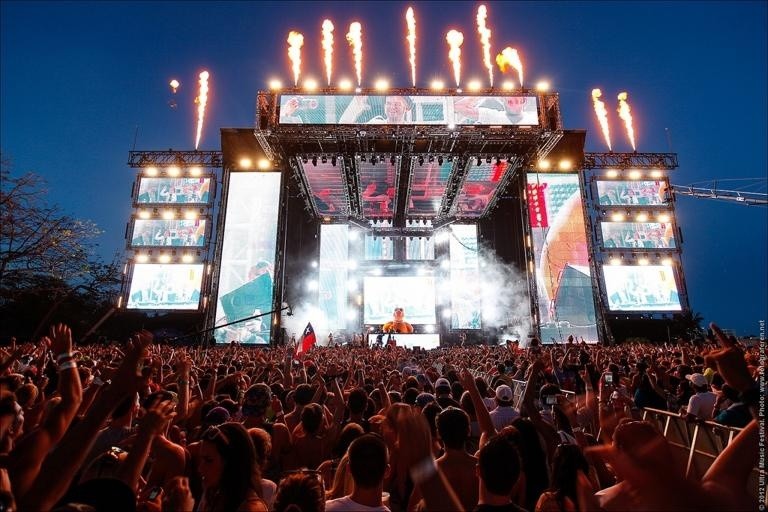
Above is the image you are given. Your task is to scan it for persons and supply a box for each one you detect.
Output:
[138,182,200,203]
[382,308,414,333]
[604,229,668,249]
[132,224,196,245]
[339,96,414,124]
[1,322,766,510]
[599,189,662,205]
[279,98,303,124]
[363,163,396,211]
[453,96,537,125]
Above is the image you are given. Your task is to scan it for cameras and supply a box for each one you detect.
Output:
[604,372,613,384]
[148,488,161,501]
[110,446,128,460]
[293,360,300,365]
[144,390,179,412]
[545,395,558,405]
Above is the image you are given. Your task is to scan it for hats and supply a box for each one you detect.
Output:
[435,377,451,390]
[685,373,708,388]
[495,383,513,402]
